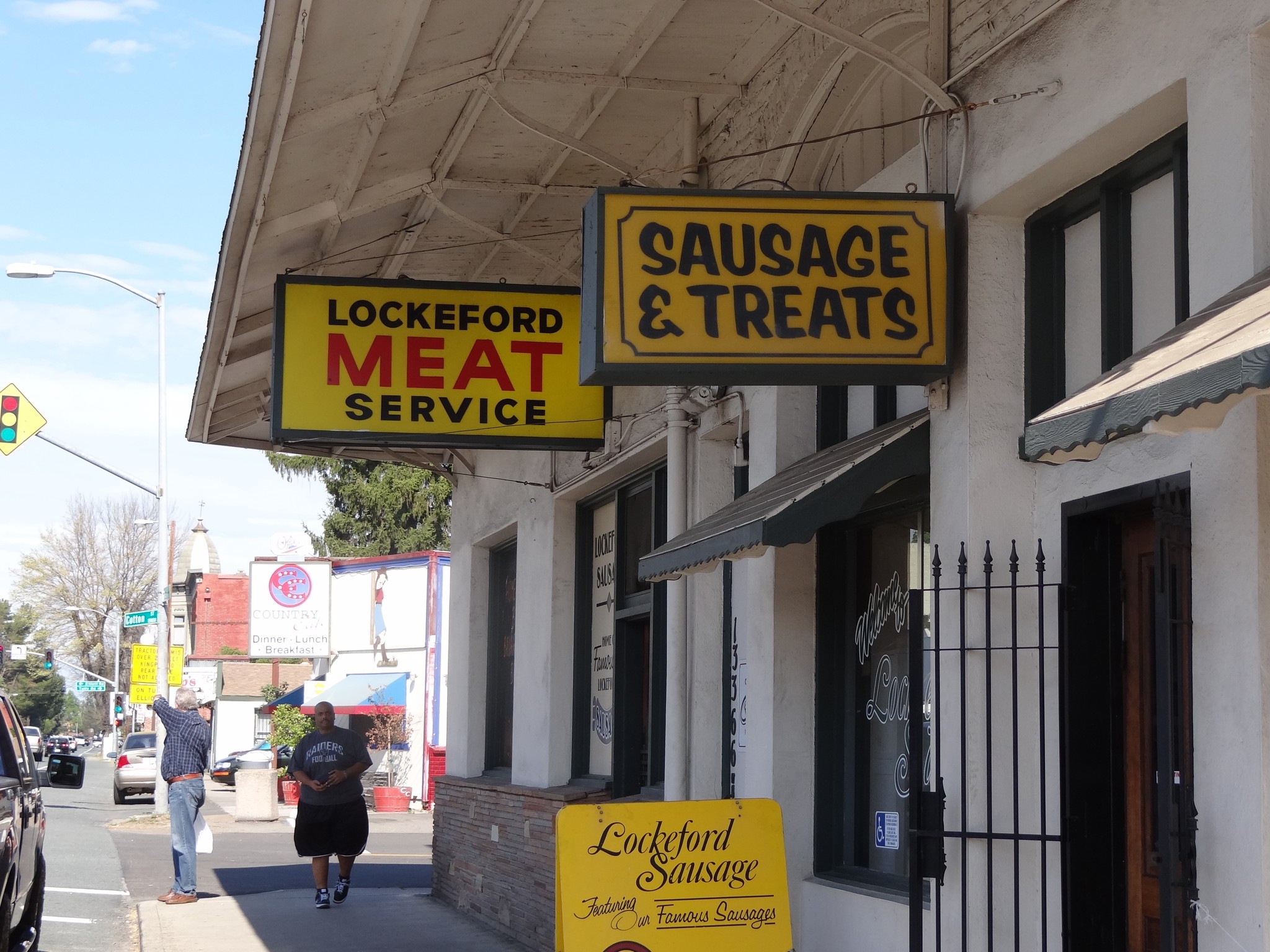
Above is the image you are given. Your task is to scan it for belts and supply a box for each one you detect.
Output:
[167,773,203,784]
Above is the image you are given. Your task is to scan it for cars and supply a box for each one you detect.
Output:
[44,735,102,757]
[106,730,157,804]
[208,731,295,786]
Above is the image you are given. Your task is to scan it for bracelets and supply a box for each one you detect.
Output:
[343,770,347,779]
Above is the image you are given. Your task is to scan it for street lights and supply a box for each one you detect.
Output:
[64,607,121,763]
[6,262,172,820]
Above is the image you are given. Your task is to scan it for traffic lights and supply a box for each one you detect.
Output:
[44,649,53,671]
[114,719,123,727]
[0,645,5,665]
[114,694,125,719]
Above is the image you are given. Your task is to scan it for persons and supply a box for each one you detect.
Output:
[285,701,373,908]
[152,688,211,904]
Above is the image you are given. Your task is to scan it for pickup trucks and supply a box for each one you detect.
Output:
[23,725,48,761]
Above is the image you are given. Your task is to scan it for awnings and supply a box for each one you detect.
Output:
[1025,267,1270,468]
[639,405,930,583]
[262,675,325,714]
[301,673,407,714]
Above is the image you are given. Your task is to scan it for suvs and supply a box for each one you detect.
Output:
[0,687,86,952]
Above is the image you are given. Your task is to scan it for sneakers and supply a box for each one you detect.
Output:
[333,877,351,904]
[315,887,330,909]
[165,893,198,904]
[158,888,176,901]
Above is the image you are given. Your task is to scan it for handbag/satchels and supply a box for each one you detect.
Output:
[193,811,213,854]
